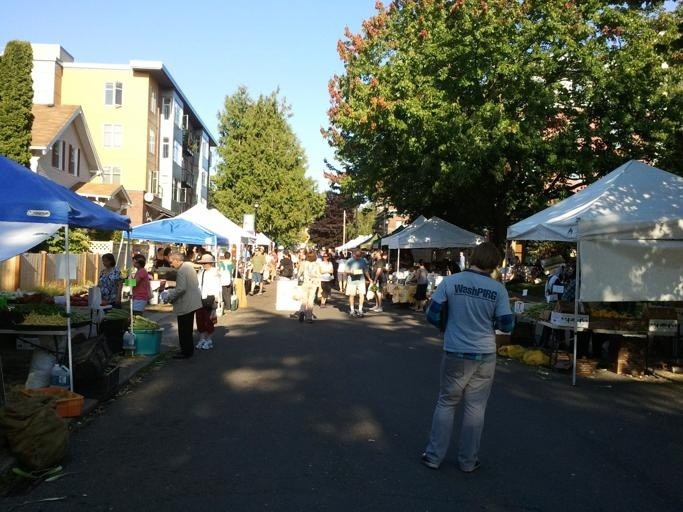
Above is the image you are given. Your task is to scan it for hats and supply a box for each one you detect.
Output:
[197,254,218,264]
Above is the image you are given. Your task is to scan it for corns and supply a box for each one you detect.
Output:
[128,315,159,330]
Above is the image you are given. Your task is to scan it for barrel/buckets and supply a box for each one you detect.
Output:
[646,331,676,369]
[616,333,647,376]
[591,328,617,370]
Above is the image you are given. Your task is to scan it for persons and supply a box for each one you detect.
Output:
[403,251,461,311]
[97,243,389,358]
[545,264,575,343]
[419,241,522,474]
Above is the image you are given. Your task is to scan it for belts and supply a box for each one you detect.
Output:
[446,350,495,358]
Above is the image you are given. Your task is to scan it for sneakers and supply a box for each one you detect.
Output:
[368,306,378,310]
[460,456,481,472]
[172,355,189,359]
[202,338,214,350]
[195,338,207,349]
[374,308,384,312]
[418,452,441,469]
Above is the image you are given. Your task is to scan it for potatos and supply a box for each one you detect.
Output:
[68,294,109,306]
[15,293,55,304]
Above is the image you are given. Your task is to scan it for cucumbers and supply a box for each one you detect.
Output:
[100,309,130,321]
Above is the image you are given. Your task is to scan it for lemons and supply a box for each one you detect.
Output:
[591,309,624,318]
